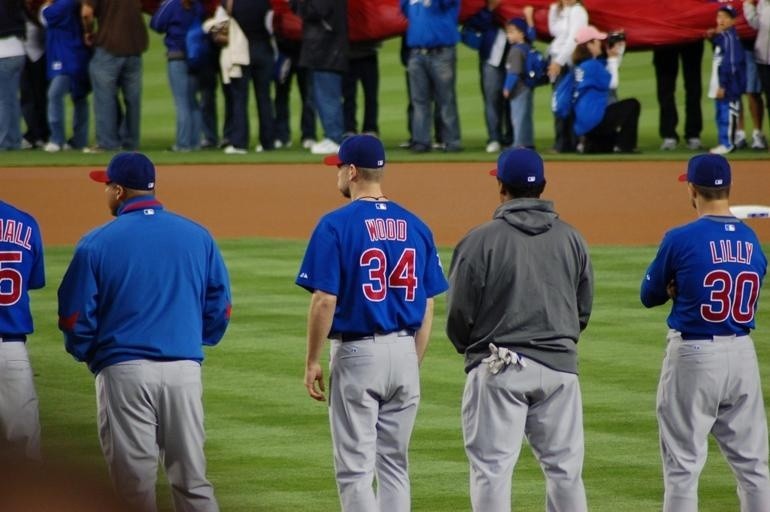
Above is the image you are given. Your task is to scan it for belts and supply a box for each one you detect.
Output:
[680,333,749,340]
[342,329,415,341]
[3,335,26,341]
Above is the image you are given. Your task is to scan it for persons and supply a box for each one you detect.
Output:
[0,198,43,470]
[1,1,770,155]
[295,134,448,511]
[500,18,536,149]
[573,26,641,155]
[448,146,593,510]
[706,5,764,153]
[58,153,231,511]
[639,154,770,510]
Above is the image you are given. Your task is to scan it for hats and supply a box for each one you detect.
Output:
[489,148,543,184]
[679,154,730,186]
[719,5,737,18]
[90,153,155,190]
[324,135,385,168]
[576,26,608,45]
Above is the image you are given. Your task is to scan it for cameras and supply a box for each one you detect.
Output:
[607,32,626,48]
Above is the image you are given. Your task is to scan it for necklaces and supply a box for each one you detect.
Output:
[353,193,391,202]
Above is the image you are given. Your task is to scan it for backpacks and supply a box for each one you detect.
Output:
[552,70,578,117]
[517,45,548,85]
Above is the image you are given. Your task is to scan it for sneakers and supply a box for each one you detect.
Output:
[303,138,340,155]
[486,141,500,152]
[660,131,765,154]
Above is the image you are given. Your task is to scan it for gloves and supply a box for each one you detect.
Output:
[481,343,526,374]
